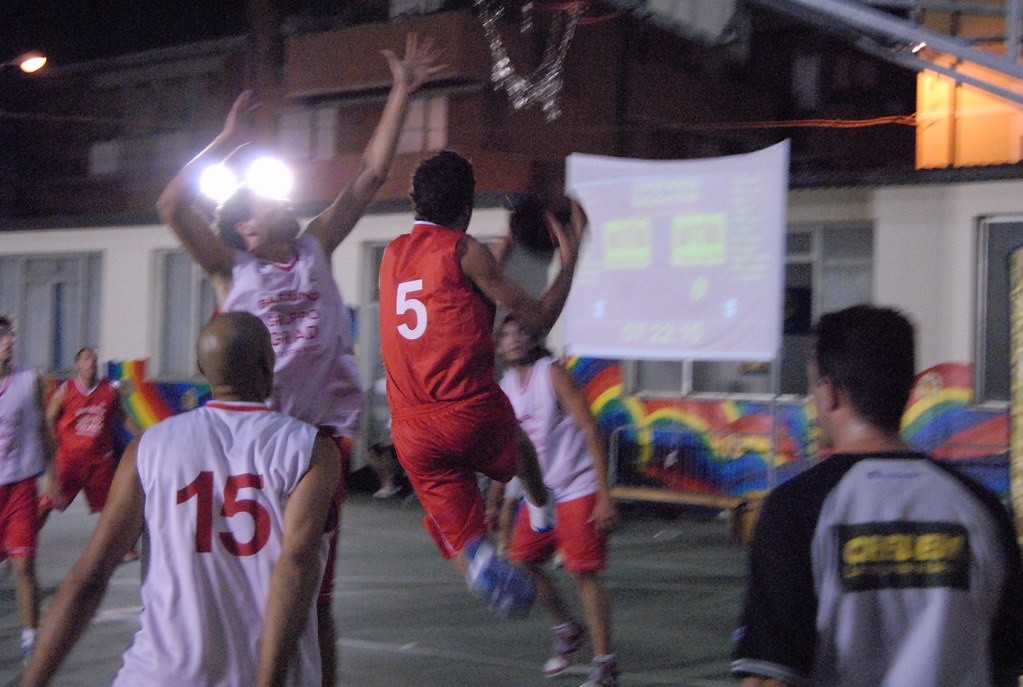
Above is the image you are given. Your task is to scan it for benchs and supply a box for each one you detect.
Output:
[610,484,764,550]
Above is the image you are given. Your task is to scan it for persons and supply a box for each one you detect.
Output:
[16,310,343,687]
[730,303,1023,687]
[35,347,142,563]
[155,29,449,686]
[372,149,620,686]
[0,316,50,668]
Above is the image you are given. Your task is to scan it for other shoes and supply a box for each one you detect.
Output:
[373,484,403,499]
[121,547,139,562]
[23,642,35,666]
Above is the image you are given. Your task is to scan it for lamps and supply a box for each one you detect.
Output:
[0,46,49,82]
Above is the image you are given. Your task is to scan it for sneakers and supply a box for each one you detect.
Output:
[464,546,535,618]
[577,653,619,687]
[541,624,588,677]
[524,486,556,532]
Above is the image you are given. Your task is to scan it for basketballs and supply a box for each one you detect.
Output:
[510,189,585,258]
